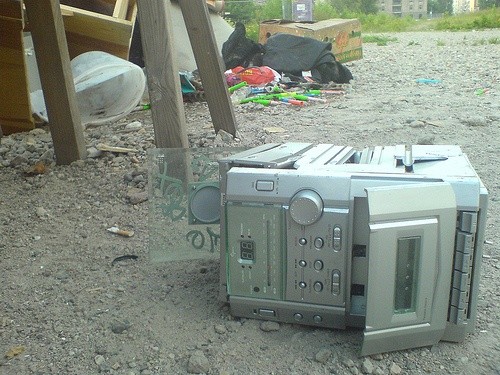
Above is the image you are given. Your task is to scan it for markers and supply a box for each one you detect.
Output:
[136,68,349,108]
[417,78,440,83]
[476,88,490,95]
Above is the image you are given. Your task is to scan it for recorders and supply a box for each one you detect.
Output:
[145,138,488,350]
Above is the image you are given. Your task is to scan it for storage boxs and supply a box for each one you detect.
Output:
[258,18,363,64]
